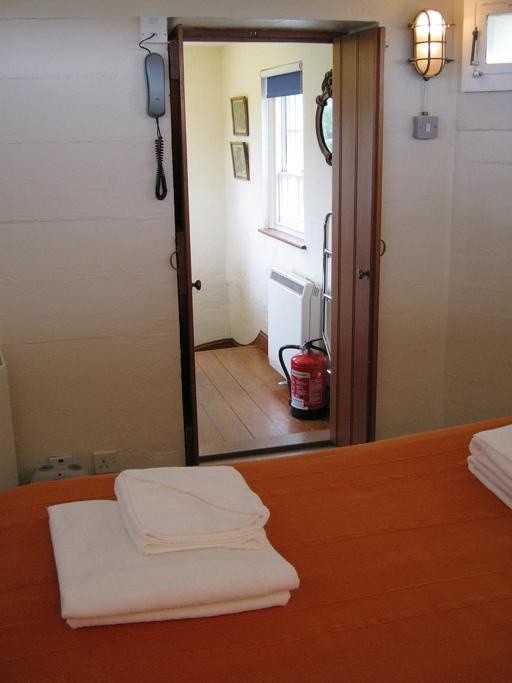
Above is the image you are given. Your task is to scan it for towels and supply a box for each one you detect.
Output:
[464,419,512,516]
[43,494,300,629]
[114,460,272,552]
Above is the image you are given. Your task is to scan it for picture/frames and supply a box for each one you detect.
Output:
[229,140,250,182]
[229,94,249,136]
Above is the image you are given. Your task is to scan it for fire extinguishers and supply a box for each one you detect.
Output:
[279,338,328,421]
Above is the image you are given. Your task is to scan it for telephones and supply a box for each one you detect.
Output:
[146,53,165,117]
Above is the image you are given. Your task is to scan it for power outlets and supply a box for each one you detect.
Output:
[93,449,119,474]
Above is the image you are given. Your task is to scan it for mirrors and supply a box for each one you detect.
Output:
[315,68,333,167]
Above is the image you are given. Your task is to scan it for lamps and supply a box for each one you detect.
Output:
[405,7,456,81]
